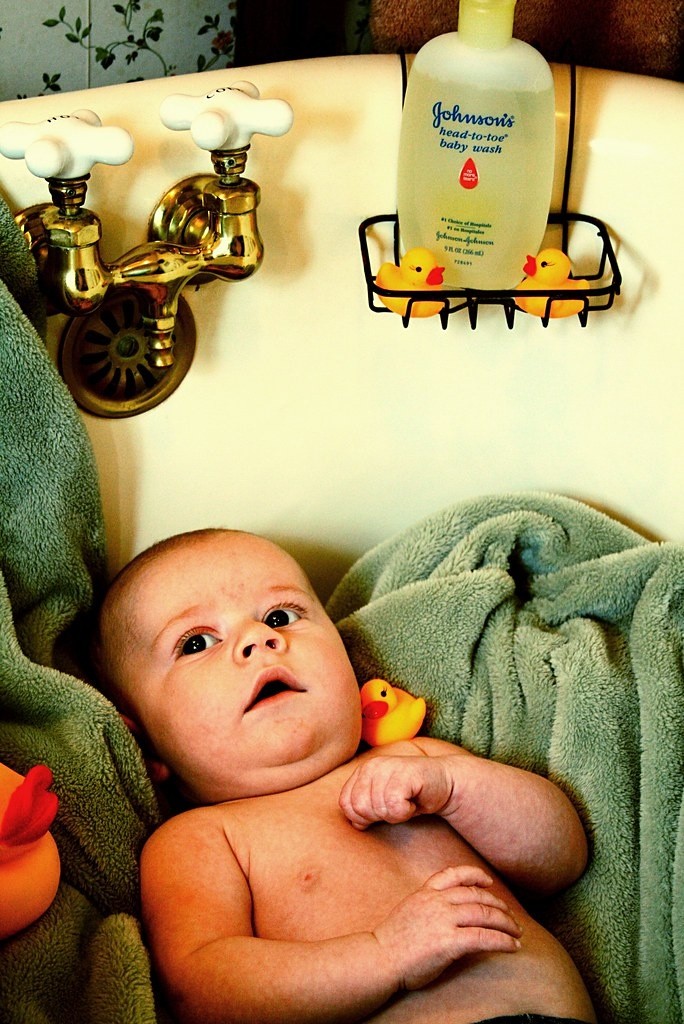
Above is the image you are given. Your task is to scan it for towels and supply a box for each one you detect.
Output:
[0,189,683,1024]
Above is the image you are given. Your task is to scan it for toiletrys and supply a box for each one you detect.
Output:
[394,0,557,291]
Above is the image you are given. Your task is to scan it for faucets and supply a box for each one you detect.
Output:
[0,79,296,371]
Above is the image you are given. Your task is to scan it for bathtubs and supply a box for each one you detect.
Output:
[0,51,684,608]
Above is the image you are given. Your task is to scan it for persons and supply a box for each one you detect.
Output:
[98,530,592,1024]
